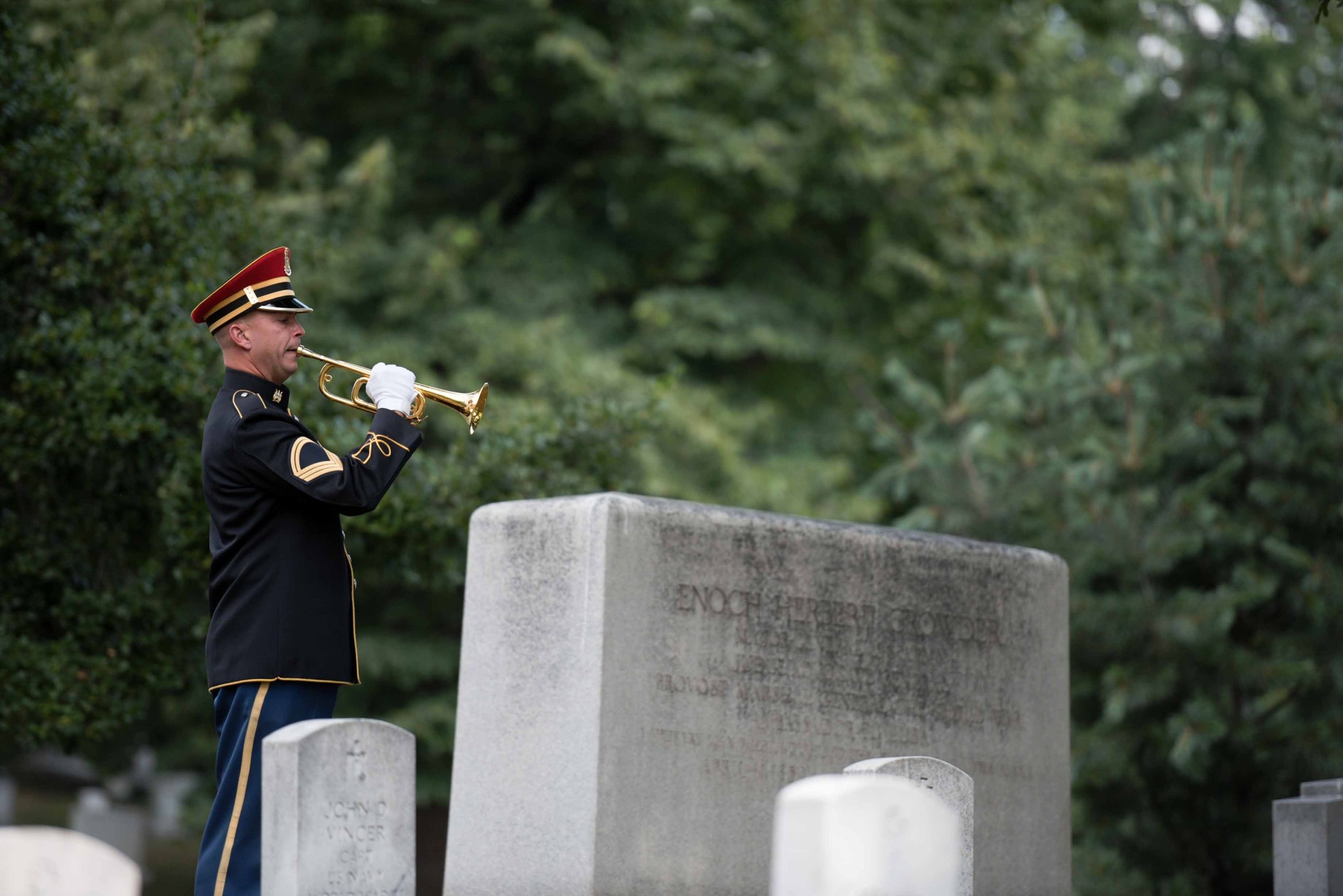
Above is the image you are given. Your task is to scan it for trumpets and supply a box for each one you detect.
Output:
[296,344,489,435]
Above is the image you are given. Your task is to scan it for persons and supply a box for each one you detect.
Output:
[192,245,423,896]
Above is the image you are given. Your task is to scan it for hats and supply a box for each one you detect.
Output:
[191,245,315,336]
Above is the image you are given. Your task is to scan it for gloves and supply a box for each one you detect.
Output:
[364,362,418,421]
